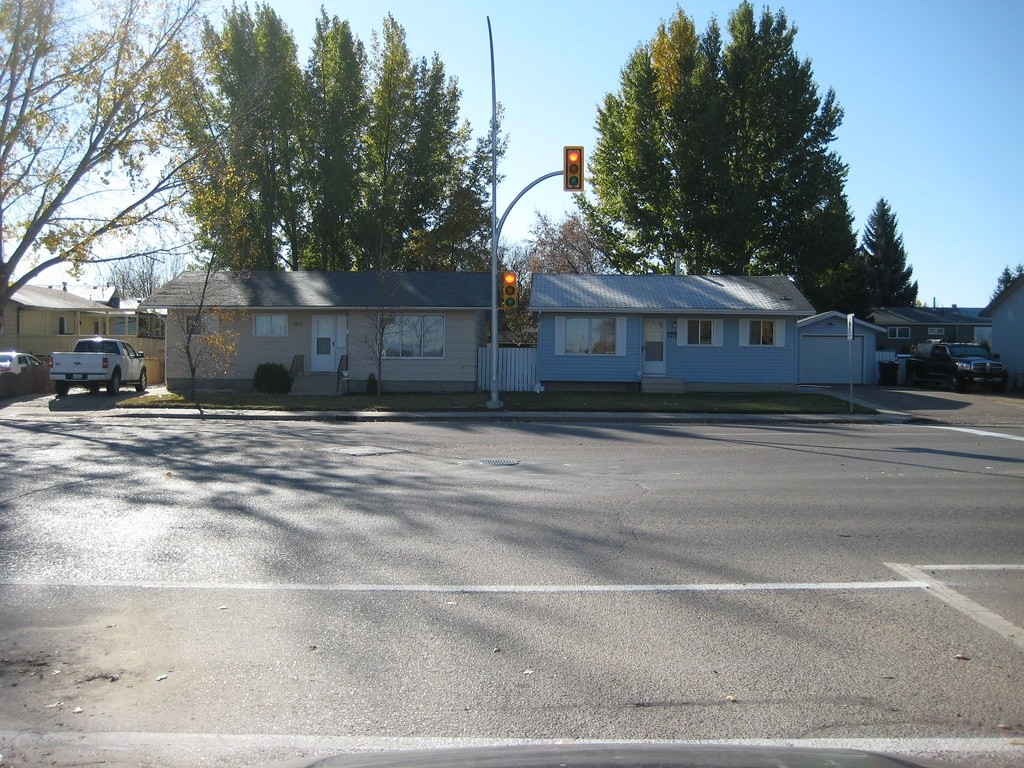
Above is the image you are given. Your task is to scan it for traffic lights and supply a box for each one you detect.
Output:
[503,272,517,309]
[564,146,584,191]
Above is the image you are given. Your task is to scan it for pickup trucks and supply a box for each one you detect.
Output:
[915,342,1010,392]
[48,338,147,395]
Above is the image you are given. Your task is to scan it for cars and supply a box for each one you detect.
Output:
[0,352,45,375]
